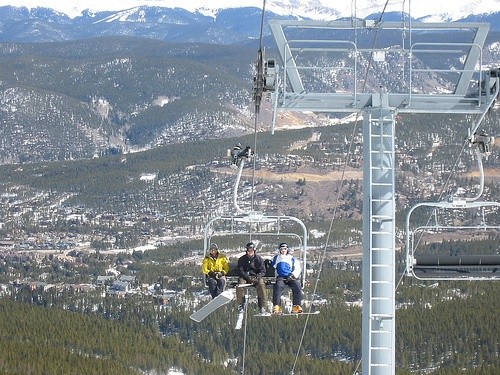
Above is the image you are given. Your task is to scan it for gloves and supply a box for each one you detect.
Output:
[209,271,224,279]
[286,275,295,283]
[249,278,258,285]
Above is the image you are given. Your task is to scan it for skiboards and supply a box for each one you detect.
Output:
[233,298,270,330]
[254,310,320,317]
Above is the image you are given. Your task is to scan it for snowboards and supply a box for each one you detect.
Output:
[189,290,234,322]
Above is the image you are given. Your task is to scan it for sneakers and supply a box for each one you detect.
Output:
[259,307,266,315]
[292,305,302,314]
[273,305,281,314]
[238,304,244,313]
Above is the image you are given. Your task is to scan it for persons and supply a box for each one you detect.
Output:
[236,242,266,314]
[272,242,302,313]
[202,243,229,299]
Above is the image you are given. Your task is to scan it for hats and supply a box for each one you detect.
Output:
[245,243,255,249]
[210,243,218,250]
[279,243,288,250]
